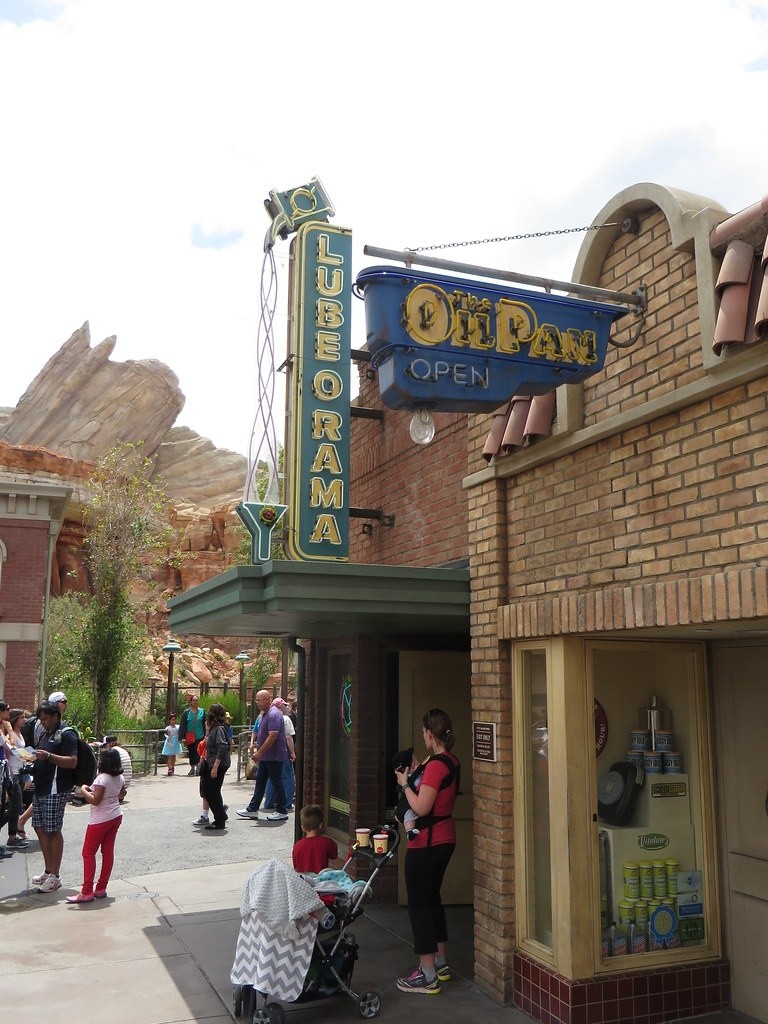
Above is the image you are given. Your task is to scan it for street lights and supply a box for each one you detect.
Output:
[235,651,251,725]
[162,638,182,725]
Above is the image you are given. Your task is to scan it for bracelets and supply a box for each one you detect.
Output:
[46,751,51,762]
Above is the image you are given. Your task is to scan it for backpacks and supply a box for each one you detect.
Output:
[21,717,46,750]
[59,727,97,792]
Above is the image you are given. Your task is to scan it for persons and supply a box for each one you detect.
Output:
[178,694,206,775]
[288,699,296,781]
[292,804,338,918]
[66,748,127,903]
[236,689,289,821]
[161,711,183,775]
[249,715,261,754]
[191,702,233,829]
[99,735,132,803]
[392,708,461,993]
[264,698,296,811]
[0,691,78,894]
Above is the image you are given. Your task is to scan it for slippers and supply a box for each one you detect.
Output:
[18,829,28,838]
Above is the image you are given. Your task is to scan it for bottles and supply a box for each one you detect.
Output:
[624,695,681,775]
[355,828,371,849]
[372,834,389,857]
[618,859,680,930]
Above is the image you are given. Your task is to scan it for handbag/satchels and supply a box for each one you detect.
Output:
[185,732,196,746]
[195,756,207,776]
[197,739,208,759]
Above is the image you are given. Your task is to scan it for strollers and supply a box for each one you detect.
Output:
[232,822,400,1024]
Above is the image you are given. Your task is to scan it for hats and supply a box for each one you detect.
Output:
[394,747,415,773]
[48,691,68,703]
[100,736,117,748]
[271,698,290,708]
[225,712,233,720]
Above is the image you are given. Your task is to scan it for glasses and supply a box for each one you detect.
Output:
[7,706,11,711]
[20,716,25,719]
[57,699,67,704]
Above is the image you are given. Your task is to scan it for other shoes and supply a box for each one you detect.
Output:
[72,798,83,807]
[203,812,228,829]
[0,839,30,859]
[188,768,195,775]
[167,768,174,775]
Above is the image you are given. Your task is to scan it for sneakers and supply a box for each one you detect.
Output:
[193,801,295,824]
[36,873,62,892]
[396,962,450,995]
[32,873,48,884]
[94,889,107,898]
[66,892,94,903]
